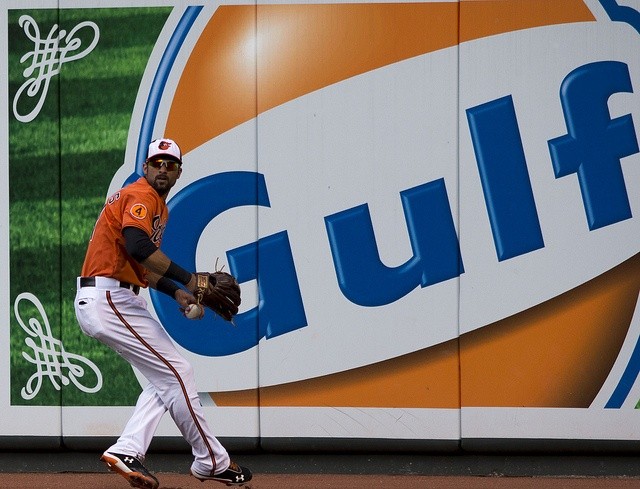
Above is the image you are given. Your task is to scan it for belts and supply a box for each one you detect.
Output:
[80,277,140,296]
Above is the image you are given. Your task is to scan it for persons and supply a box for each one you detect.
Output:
[74,137,255,488]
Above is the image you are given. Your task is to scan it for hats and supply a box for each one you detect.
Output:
[147,139,182,163]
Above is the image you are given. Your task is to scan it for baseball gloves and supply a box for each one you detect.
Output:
[196,273,241,322]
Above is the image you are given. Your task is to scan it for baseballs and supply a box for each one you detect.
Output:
[186,304,201,320]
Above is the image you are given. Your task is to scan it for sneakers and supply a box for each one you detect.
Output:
[189,461,252,486]
[101,450,159,488]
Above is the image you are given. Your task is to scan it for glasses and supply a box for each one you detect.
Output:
[147,159,180,171]
